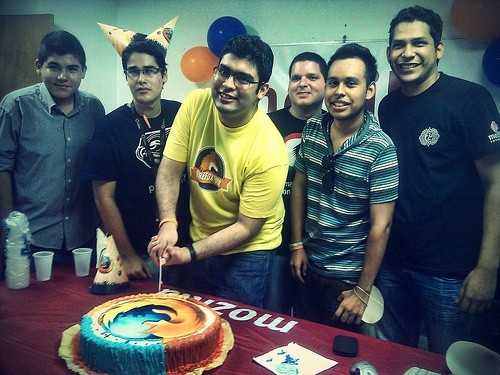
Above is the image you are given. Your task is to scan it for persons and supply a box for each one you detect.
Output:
[258,51,331,316]
[146,33,288,314]
[289,41,400,343]
[0,30,118,281]
[93,15,194,284]
[369,4,500,363]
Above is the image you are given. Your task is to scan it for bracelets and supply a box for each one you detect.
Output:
[185,242,196,263]
[158,217,179,229]
[289,242,303,248]
[290,246,305,251]
[352,288,369,307]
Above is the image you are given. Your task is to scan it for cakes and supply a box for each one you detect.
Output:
[79,293,225,375]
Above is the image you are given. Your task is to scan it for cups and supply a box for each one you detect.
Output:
[5,211,30,289]
[72,248,93,277]
[32,251,54,282]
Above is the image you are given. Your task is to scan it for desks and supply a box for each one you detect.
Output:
[0,267,451,375]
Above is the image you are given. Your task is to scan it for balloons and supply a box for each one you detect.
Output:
[451,0,500,43]
[180,46,219,82]
[482,39,500,87]
[207,16,248,57]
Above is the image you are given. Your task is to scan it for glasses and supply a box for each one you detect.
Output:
[124,66,164,77]
[321,153,336,195]
[212,65,265,90]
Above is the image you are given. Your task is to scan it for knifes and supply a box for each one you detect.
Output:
[158,254,162,293]
